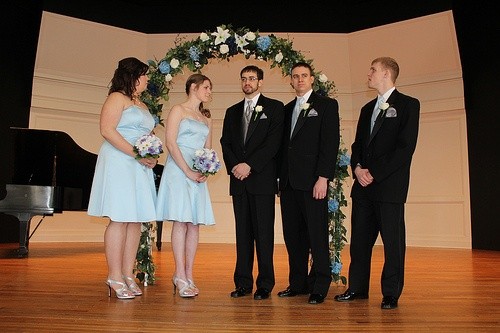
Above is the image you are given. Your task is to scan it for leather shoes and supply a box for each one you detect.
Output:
[334,288,369,302]
[231,286,252,297]
[381,297,398,309]
[278,287,310,297]
[254,288,269,300]
[308,292,324,304]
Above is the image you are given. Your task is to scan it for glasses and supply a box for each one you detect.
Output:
[239,77,258,82]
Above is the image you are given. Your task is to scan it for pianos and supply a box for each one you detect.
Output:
[0,127,164,259]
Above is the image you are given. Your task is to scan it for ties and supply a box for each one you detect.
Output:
[290,97,303,139]
[371,96,384,135]
[245,100,253,123]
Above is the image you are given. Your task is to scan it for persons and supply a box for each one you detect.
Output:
[277,63,339,304]
[88,57,158,299]
[219,65,285,300]
[158,74,213,298]
[334,57,420,309]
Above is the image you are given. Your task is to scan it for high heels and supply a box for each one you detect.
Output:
[105,273,143,299]
[172,271,199,297]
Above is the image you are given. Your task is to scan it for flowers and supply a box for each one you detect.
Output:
[376,102,394,117]
[299,101,314,116]
[191,148,221,176]
[253,105,264,121]
[131,135,164,161]
